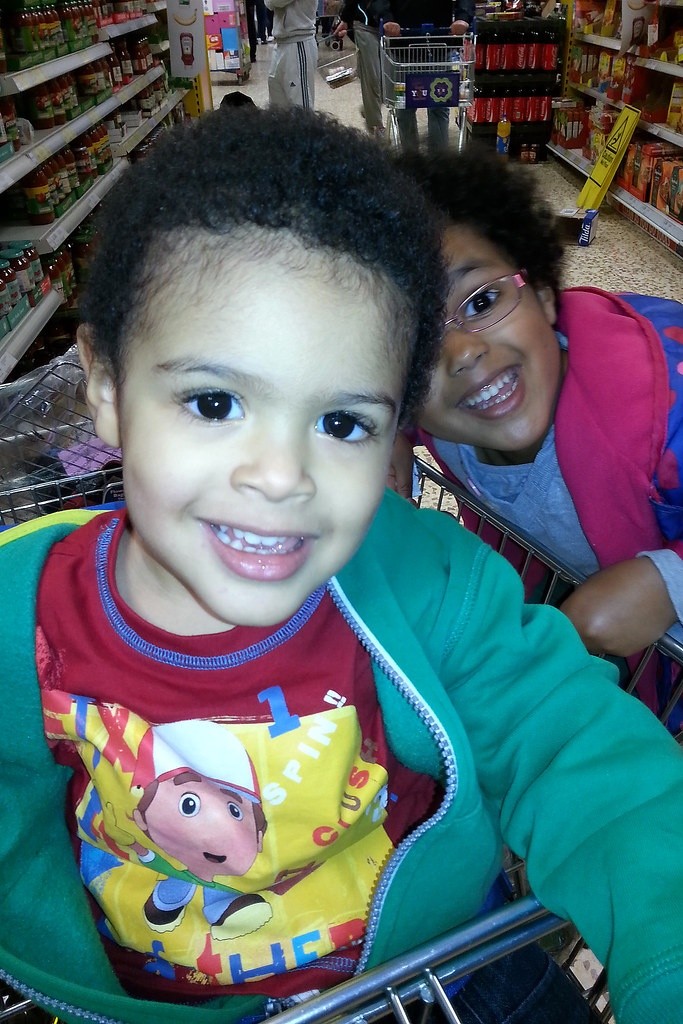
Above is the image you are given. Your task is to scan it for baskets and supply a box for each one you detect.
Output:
[314,29,359,88]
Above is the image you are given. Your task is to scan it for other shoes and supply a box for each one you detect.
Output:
[268,36,273,41]
[251,58,256,62]
[261,40,267,45]
[369,123,386,137]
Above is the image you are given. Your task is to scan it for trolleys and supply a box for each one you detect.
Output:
[378,17,477,173]
[0,352,681,1024]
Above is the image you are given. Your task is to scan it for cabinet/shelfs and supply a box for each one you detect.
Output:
[0,1,188,385]
[545,0,683,262]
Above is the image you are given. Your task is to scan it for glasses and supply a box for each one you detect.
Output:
[440,268,529,333]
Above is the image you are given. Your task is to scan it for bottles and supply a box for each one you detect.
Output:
[0,0,192,318]
[460,17,561,164]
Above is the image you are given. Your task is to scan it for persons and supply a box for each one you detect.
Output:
[0,105,683,1024]
[244,0,476,152]
